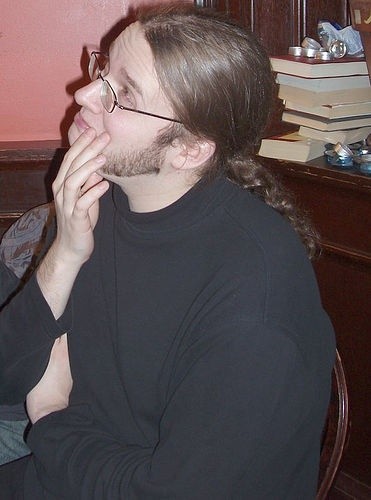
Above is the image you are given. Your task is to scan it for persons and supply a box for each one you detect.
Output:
[0,12,336,500]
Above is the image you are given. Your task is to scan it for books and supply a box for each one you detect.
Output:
[259,45,371,162]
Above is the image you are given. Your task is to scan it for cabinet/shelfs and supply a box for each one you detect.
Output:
[197,0,371,500]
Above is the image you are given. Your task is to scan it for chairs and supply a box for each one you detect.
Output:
[0,200,349,500]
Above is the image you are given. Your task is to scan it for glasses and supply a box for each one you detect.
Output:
[87,51,186,125]
